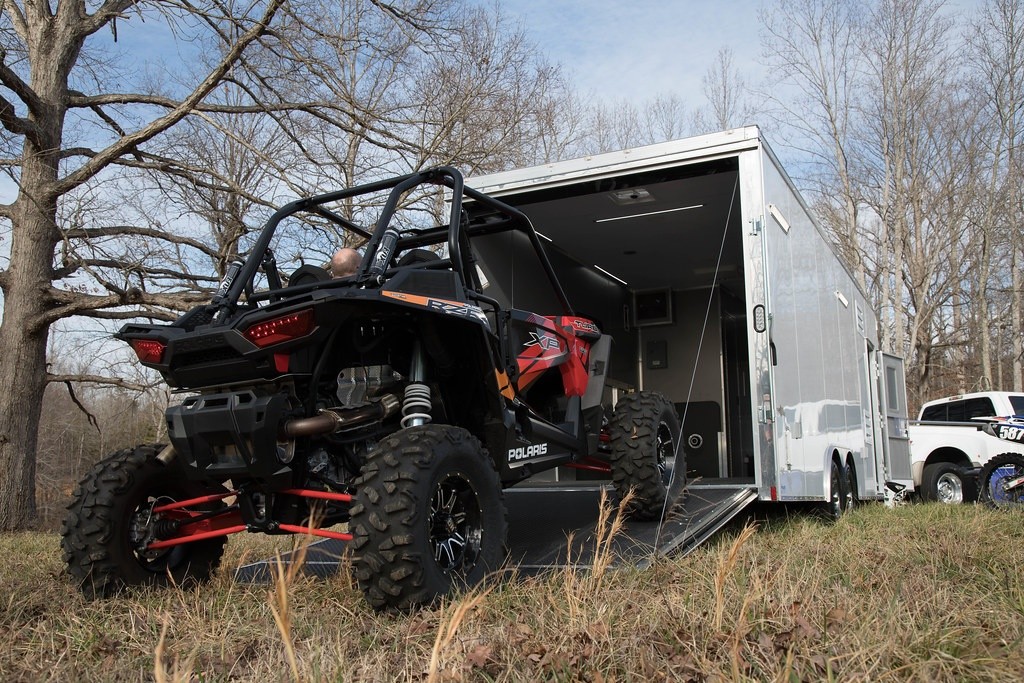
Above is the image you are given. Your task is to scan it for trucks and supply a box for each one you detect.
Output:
[441,122,913,573]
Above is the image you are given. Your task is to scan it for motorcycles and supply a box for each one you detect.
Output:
[59,165,687,621]
[972,415,1024,509]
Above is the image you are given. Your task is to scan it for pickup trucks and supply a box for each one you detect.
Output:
[907,391,1024,505]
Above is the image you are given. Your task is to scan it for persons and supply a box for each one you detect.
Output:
[329,247,363,281]
